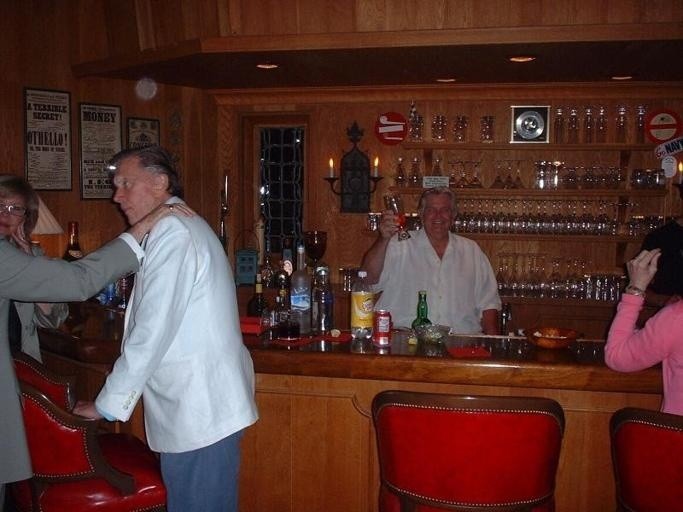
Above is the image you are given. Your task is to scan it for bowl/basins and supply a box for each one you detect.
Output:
[523,327,578,351]
[414,324,451,345]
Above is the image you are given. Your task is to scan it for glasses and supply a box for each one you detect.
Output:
[0,202,28,218]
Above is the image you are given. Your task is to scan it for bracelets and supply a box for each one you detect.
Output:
[625,285,647,299]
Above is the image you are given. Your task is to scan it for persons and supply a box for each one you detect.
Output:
[72,143,260,512]
[0,203,197,511]
[359,186,502,337]
[0,173,69,364]
[604,221,682,415]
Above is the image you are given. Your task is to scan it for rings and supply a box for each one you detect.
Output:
[168,204,176,212]
[20,236,25,241]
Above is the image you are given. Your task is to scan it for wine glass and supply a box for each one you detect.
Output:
[396,159,619,188]
[498,250,591,299]
[454,198,660,235]
[386,192,409,244]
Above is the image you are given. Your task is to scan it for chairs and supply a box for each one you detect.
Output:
[371,389,567,512]
[7,350,167,512]
[609,408,681,511]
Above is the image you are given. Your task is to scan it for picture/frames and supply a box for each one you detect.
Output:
[126,118,161,151]
[78,102,123,201]
[23,86,71,191]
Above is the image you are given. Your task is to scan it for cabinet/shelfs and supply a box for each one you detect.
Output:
[394,101,668,275]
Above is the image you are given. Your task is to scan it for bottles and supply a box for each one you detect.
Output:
[372,310,392,349]
[634,106,647,144]
[274,260,289,287]
[615,107,628,142]
[258,318,272,348]
[62,221,83,262]
[583,106,595,144]
[567,107,580,144]
[248,274,268,316]
[413,291,432,332]
[554,108,564,144]
[290,244,311,314]
[350,271,374,339]
[312,269,333,333]
[261,239,274,281]
[596,106,607,143]
